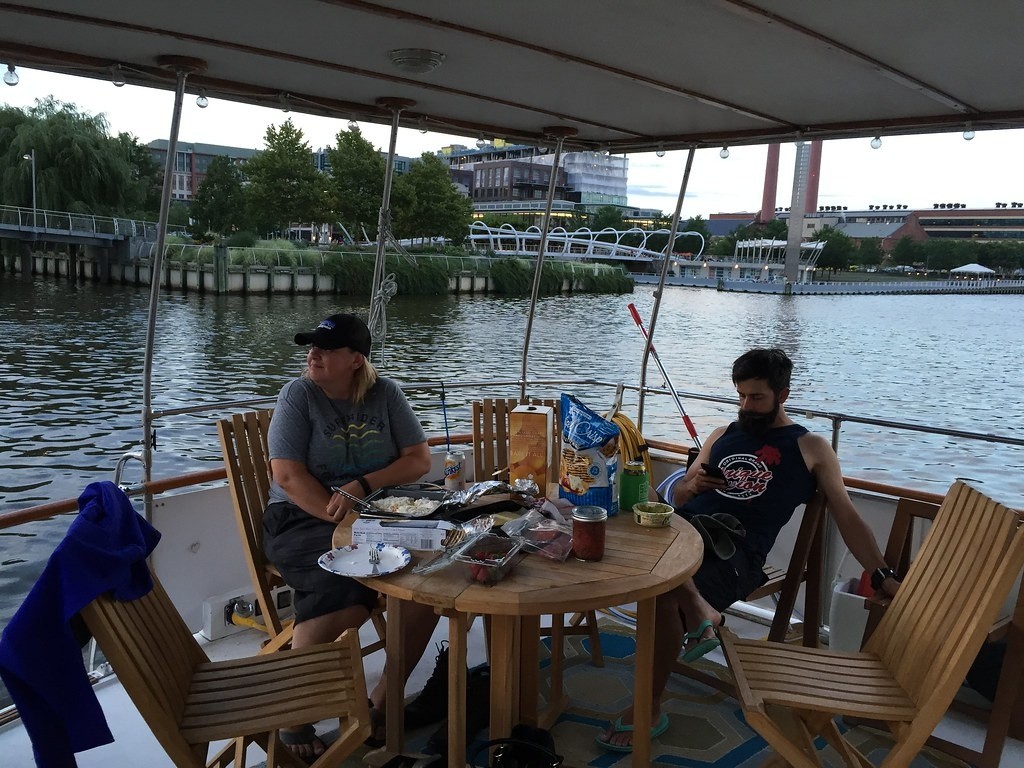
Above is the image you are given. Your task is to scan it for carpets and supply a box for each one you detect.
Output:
[250,618,973,768]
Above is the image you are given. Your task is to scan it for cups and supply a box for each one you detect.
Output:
[571,505,607,562]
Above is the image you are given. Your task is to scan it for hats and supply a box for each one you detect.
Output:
[293,314,372,357]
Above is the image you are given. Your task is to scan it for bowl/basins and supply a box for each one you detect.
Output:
[411,532,525,585]
[632,501,674,526]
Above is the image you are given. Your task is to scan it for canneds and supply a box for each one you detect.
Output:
[623,461,646,476]
[445,451,466,491]
[570,506,607,562]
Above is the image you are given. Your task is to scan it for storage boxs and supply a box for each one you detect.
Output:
[510,404,553,498]
[351,519,457,551]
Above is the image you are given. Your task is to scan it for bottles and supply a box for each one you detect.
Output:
[619,460,649,510]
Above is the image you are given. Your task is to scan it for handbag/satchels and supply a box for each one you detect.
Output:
[469,724,565,768]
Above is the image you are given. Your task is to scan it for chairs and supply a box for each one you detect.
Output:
[719,482,1024,768]
[672,450,829,703]
[54,481,373,768]
[472,396,606,703]
[842,496,1024,768]
[216,407,387,657]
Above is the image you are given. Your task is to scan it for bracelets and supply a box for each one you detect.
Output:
[871,567,897,590]
[356,476,372,497]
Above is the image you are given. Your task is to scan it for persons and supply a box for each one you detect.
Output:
[595,347,902,753]
[262,313,432,757]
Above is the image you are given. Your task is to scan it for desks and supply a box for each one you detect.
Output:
[332,482,705,768]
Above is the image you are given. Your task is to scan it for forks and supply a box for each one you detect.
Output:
[368,549,381,575]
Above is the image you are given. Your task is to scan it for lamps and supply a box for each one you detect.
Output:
[385,48,446,73]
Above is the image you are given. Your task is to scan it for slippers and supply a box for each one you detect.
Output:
[594,711,668,751]
[682,620,719,664]
[361,697,387,748]
[277,723,326,765]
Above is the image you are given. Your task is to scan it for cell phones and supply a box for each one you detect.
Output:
[700,462,729,487]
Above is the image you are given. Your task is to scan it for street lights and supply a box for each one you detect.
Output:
[23,148,36,225]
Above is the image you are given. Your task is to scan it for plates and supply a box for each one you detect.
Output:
[352,485,446,520]
[317,543,411,577]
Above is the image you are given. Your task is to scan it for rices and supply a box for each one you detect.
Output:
[371,497,442,517]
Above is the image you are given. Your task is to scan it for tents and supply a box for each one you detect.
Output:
[949,263,995,281]
